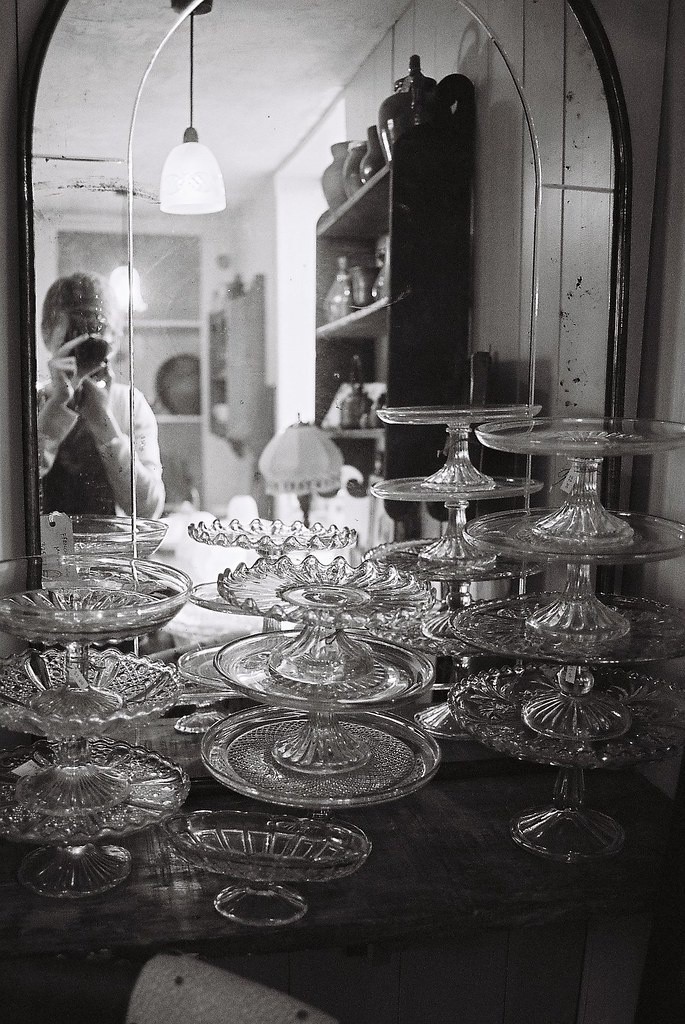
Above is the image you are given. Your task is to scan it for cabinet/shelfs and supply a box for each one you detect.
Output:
[103,318,204,518]
[210,291,261,444]
[1,759,678,1024]
[315,157,469,521]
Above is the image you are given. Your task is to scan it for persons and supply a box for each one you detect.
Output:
[36,271,165,533]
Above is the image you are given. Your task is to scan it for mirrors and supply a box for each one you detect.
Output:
[22,1,634,780]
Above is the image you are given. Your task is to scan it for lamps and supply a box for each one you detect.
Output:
[158,0,227,214]
[109,191,147,316]
[258,417,343,531]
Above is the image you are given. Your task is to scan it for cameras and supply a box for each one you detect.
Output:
[73,337,109,368]
